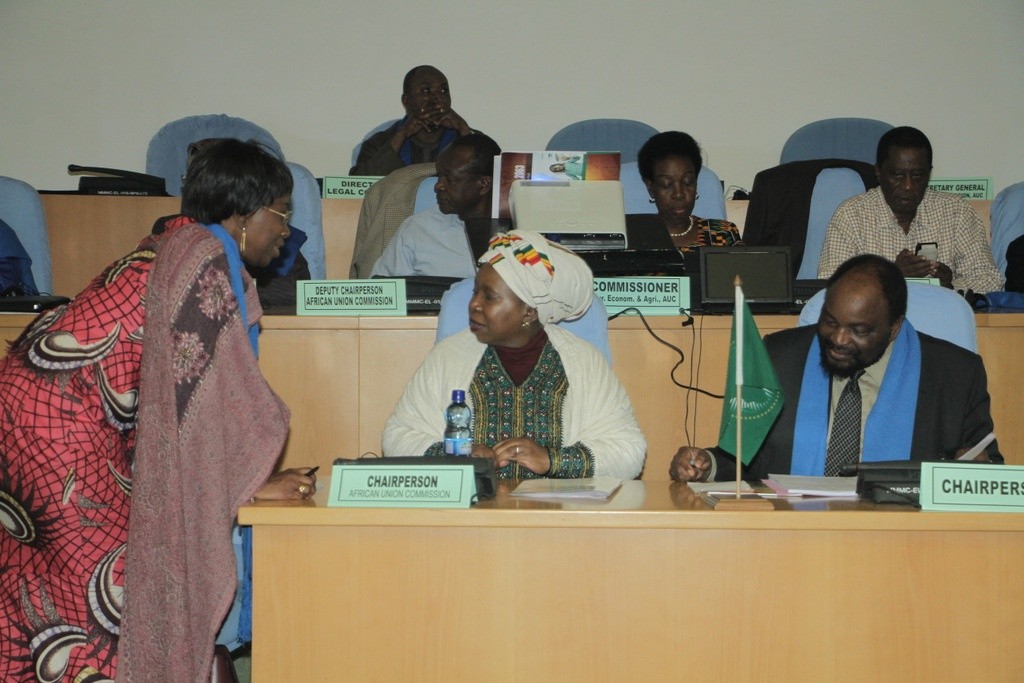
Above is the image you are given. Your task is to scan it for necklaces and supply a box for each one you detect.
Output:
[661,215,696,237]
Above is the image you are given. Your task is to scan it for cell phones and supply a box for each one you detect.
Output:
[915,242,937,267]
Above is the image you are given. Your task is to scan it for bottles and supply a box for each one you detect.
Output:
[444,390,473,458]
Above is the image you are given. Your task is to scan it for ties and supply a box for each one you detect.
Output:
[825,368,866,476]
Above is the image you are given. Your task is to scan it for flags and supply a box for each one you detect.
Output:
[717,275,784,466]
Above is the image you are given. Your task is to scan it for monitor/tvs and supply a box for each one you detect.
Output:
[509,179,627,251]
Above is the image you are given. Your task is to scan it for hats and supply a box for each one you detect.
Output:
[478,229,594,325]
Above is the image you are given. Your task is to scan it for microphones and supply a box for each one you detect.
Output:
[68,164,166,191]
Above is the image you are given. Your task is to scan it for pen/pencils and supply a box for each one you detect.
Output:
[306,466,320,476]
[689,460,696,468]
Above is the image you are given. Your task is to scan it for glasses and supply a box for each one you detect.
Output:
[260,203,294,224]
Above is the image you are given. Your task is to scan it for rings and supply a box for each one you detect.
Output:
[514,447,521,454]
[298,485,307,495]
[441,108,444,113]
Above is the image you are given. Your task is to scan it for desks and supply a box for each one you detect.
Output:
[0,190,1024,683]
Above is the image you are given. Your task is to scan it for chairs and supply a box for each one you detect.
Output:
[435,276,614,371]
[796,282,977,357]
[544,119,661,165]
[619,163,727,221]
[742,158,881,279]
[989,180,1024,289]
[777,117,896,165]
[0,176,52,296]
[350,164,439,280]
[145,115,286,197]
[281,161,326,280]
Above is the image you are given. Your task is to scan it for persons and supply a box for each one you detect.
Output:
[371,131,514,277]
[816,126,1005,294]
[668,253,1004,482]
[348,65,469,175]
[627,131,741,276]
[0,137,320,683]
[0,219,20,294]
[180,138,311,316]
[380,230,647,483]
